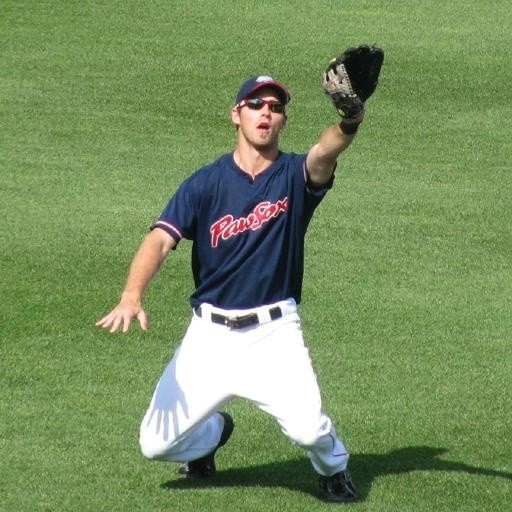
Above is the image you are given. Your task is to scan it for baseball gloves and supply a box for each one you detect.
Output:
[323,43,384,135]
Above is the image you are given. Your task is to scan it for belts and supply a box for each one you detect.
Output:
[195,305,282,328]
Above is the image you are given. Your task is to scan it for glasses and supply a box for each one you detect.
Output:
[235,98,285,113]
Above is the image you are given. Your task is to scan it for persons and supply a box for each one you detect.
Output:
[95,46,384,502]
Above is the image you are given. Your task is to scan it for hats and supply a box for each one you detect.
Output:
[237,75,290,106]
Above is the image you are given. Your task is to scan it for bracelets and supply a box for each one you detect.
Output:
[339,121,361,135]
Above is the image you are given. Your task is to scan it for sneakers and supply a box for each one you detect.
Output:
[318,469,359,501]
[187,411,234,476]
